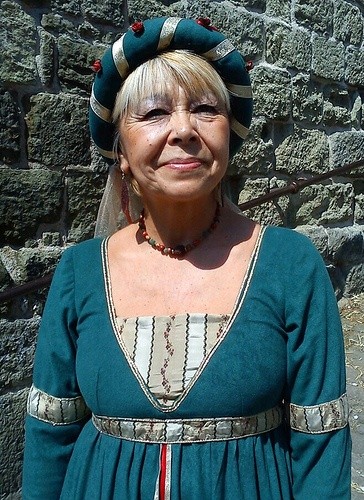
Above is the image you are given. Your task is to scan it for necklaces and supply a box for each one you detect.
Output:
[138,201,221,255]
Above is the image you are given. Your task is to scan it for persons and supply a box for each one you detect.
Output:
[20,17,351,500]
